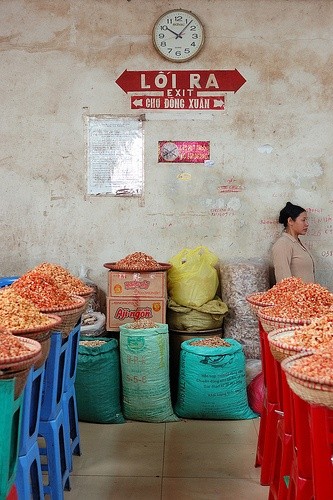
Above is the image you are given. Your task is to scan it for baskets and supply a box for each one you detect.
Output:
[280,352,333,409]
[36,294,86,339]
[8,314,62,372]
[267,325,316,363]
[257,307,312,335]
[0,336,42,400]
[75,291,93,314]
[245,292,275,311]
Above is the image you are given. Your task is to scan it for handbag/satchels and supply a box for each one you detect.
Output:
[167,245,219,308]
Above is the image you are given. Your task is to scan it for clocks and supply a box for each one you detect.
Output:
[153,9,205,63]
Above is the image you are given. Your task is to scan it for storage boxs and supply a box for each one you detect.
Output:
[105,296,167,331]
[107,270,168,297]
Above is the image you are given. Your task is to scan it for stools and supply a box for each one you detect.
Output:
[255,321,333,500]
[0,276,86,499]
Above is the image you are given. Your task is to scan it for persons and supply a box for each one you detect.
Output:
[271,202,316,284]
